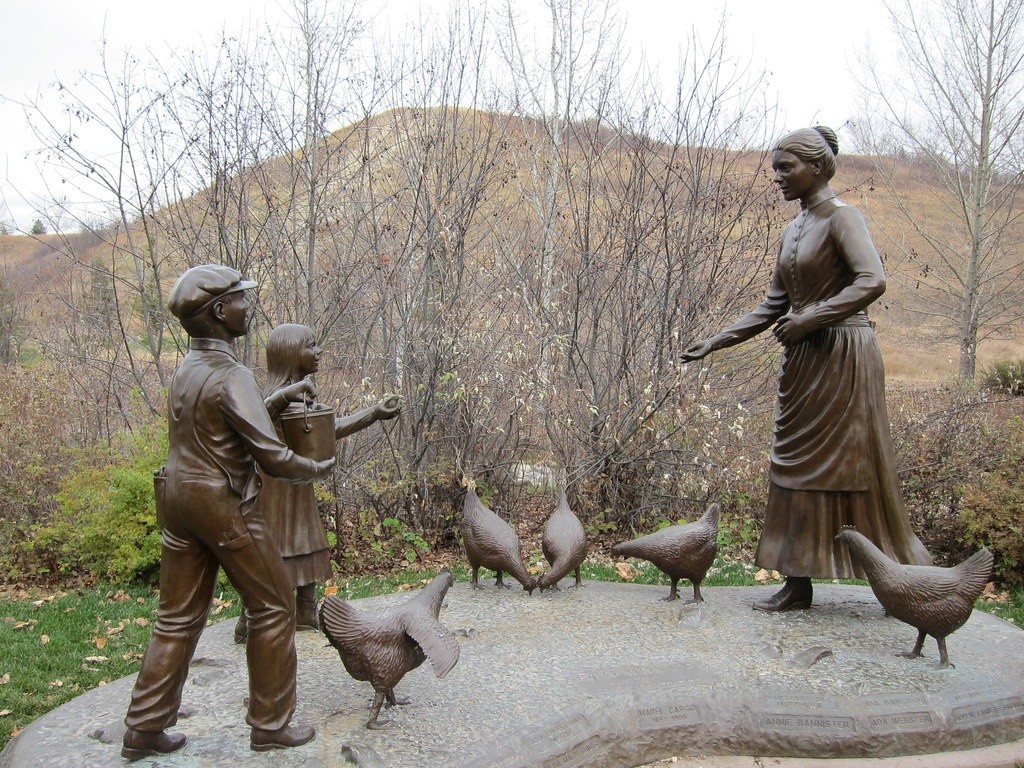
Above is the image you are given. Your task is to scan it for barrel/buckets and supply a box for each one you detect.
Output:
[280,390,338,461]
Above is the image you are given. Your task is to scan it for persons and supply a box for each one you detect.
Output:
[676,126,934,614]
[120,265,318,762]
[234,322,404,644]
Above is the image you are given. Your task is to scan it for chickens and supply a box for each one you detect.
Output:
[536,490,588,593]
[835,525,994,670]
[459,484,536,595]
[317,570,461,727]
[610,502,718,605]
